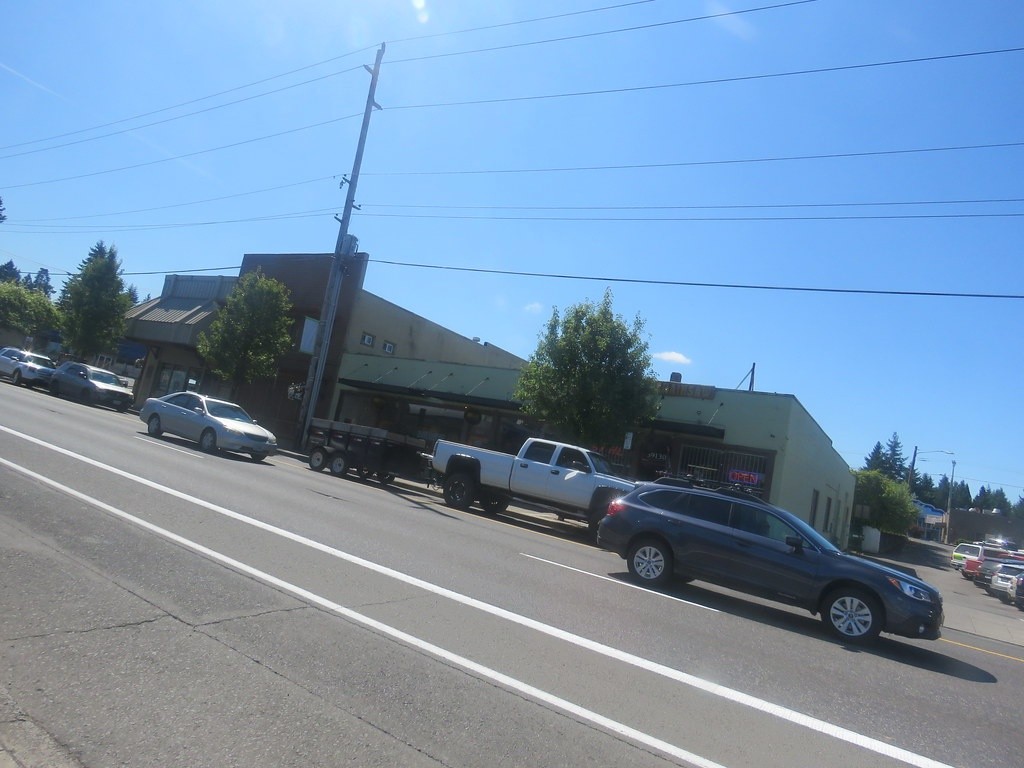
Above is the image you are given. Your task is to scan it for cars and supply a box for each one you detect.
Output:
[139,390,278,464]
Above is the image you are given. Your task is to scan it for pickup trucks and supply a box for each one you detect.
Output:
[432,434,636,535]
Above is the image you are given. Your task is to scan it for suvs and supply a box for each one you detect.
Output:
[949,537,1024,611]
[597,477,945,647]
[48,361,135,414]
[0,346,56,387]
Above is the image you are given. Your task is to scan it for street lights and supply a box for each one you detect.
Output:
[907,445,956,487]
